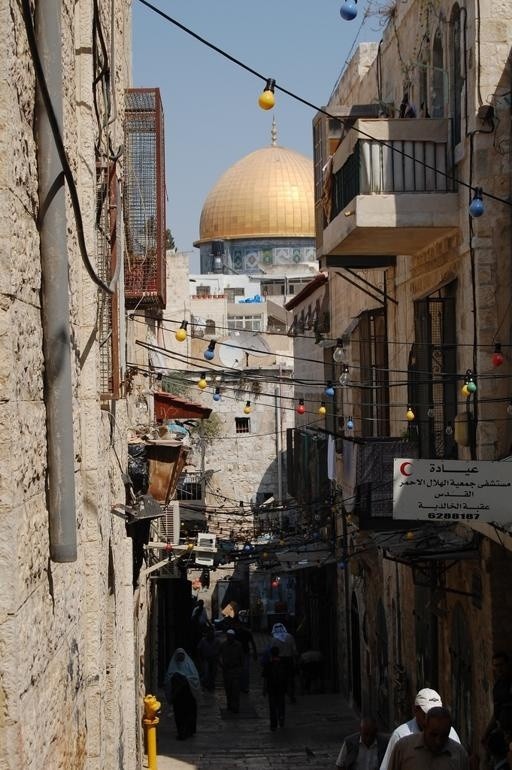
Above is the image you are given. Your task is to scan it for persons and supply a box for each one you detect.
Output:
[485,725,512,770]
[379,688,462,770]
[333,714,391,770]
[233,620,257,694]
[479,651,512,770]
[196,627,221,692]
[162,645,203,741]
[190,609,208,643]
[264,620,298,704]
[219,629,244,713]
[190,600,215,632]
[261,644,294,732]
[387,707,471,770]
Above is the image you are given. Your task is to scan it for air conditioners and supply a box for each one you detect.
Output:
[153,497,181,581]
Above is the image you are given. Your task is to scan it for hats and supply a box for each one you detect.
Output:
[414,688,442,714]
